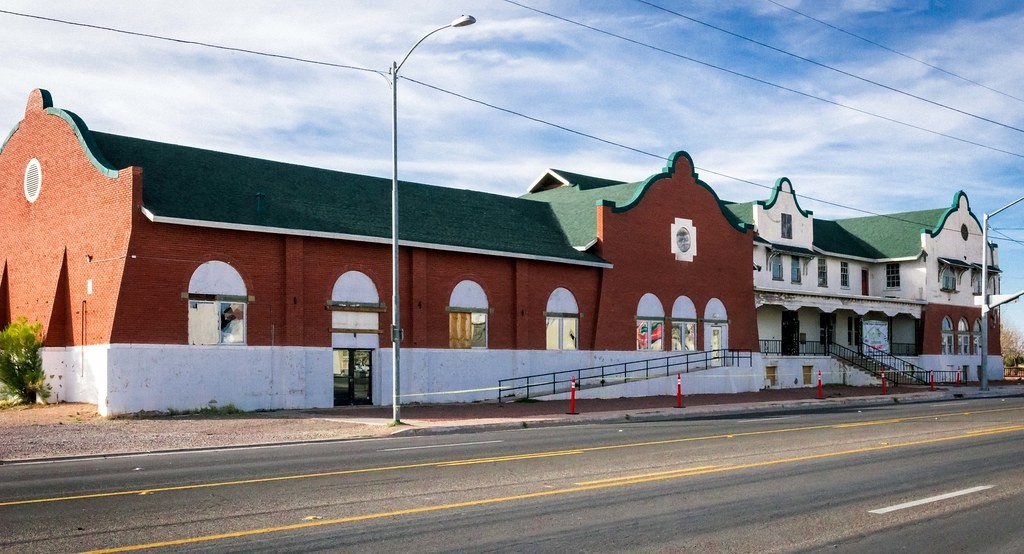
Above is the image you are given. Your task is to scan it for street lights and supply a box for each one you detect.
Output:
[390,16,476,425]
